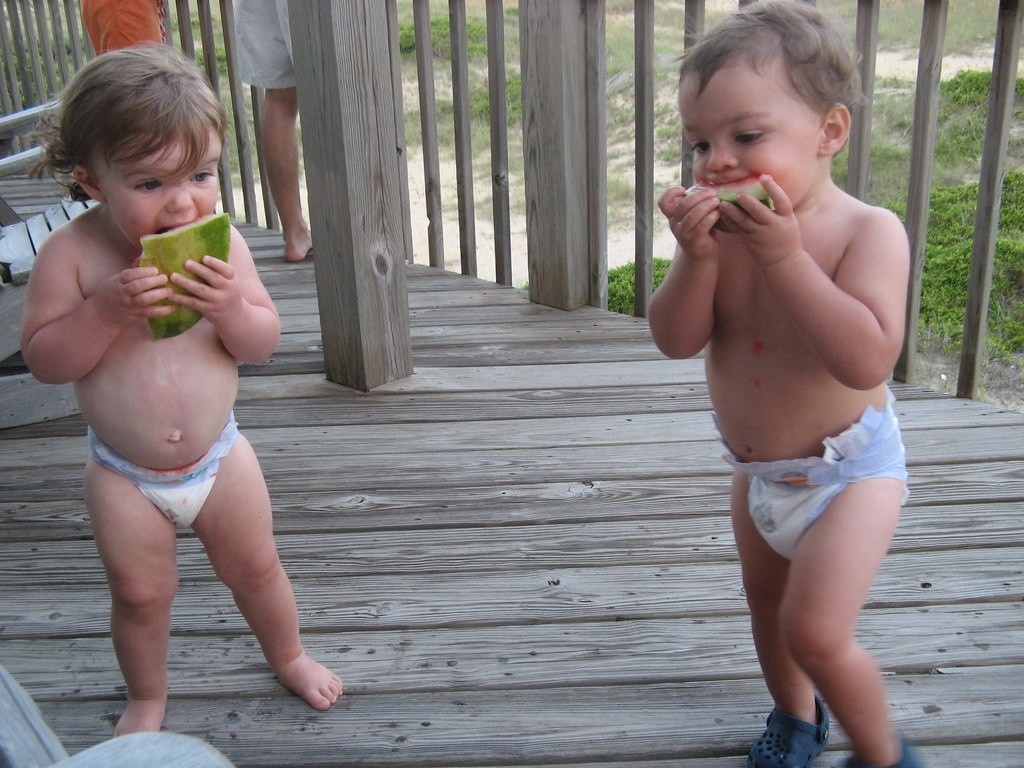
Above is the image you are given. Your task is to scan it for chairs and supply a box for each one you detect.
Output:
[0,99,102,429]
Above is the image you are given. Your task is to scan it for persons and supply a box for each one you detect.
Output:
[81,0,166,56]
[21,42,342,739]
[648,0,919,768]
[235,0,313,261]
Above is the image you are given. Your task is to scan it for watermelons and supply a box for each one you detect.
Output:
[133,212,231,339]
[684,173,777,233]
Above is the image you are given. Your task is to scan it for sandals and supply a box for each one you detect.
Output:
[831,732,920,768]
[747,696,829,768]
